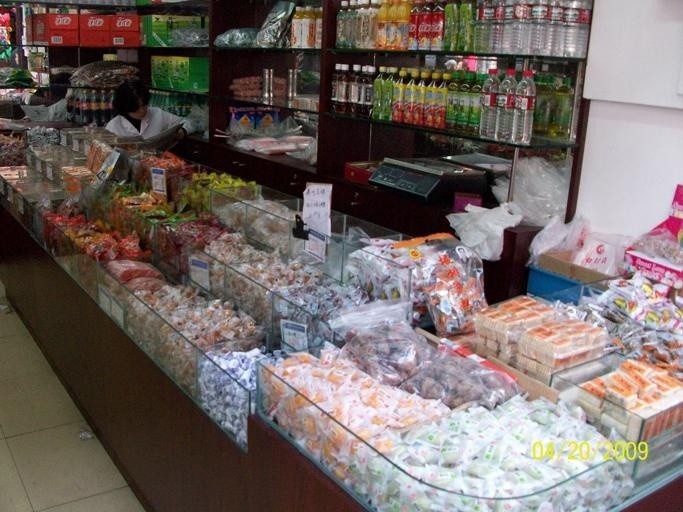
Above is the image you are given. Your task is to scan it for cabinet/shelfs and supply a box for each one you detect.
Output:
[1,0,682,512]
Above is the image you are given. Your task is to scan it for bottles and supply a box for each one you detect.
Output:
[284,67,298,99]
[62,88,117,127]
[290,4,324,49]
[333,0,594,151]
[261,67,274,105]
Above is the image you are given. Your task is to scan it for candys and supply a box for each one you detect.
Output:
[42,146,683,512]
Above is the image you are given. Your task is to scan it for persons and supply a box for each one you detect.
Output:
[102,80,198,152]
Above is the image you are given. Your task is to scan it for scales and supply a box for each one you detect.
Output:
[367,156,488,201]
